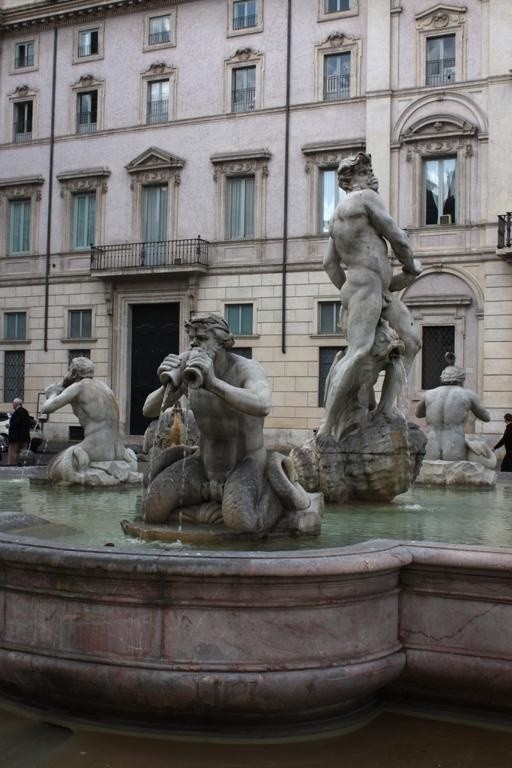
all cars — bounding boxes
[0,410,45,452]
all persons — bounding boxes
[414,364,491,461]
[315,151,424,435]
[141,311,285,533]
[40,356,137,468]
[6,397,31,465]
[491,412,512,472]
